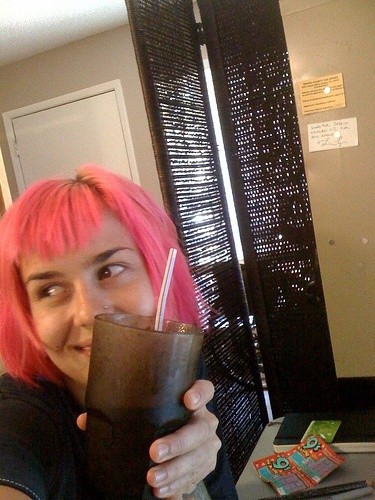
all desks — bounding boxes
[235,415,375,500]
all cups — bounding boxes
[80,313,205,500]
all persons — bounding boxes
[0,165,240,500]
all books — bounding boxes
[272,409,375,453]
